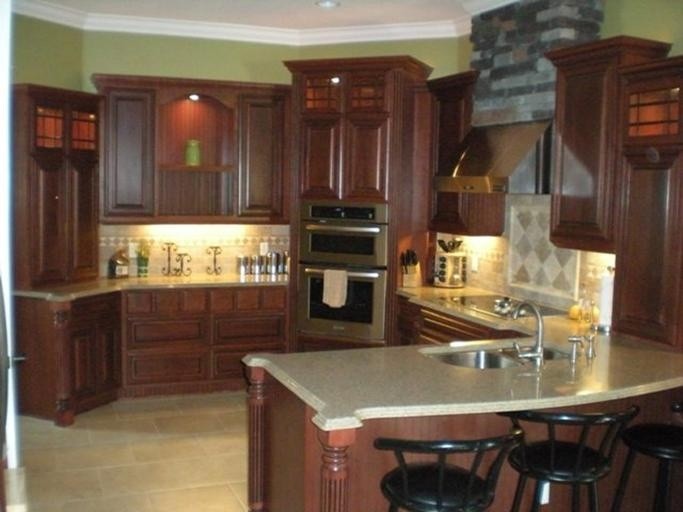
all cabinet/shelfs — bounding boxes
[236,80,286,225]
[207,288,292,397]
[607,54,683,352]
[426,71,504,238]
[123,290,205,401]
[281,53,436,204]
[155,76,236,225]
[90,72,153,225]
[14,291,123,430]
[412,307,519,347]
[549,36,675,256]
[13,82,102,292]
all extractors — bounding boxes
[430,116,556,196]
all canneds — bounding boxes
[236,251,289,274]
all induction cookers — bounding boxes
[438,293,570,321]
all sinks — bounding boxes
[501,345,579,368]
[430,348,524,370]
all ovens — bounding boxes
[294,199,389,339]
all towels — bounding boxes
[321,267,350,311]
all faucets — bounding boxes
[513,299,545,368]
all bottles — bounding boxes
[136,239,149,278]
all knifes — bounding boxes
[399,248,419,266]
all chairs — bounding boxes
[373,422,523,510]
[498,405,640,511]
[613,400,682,511]
